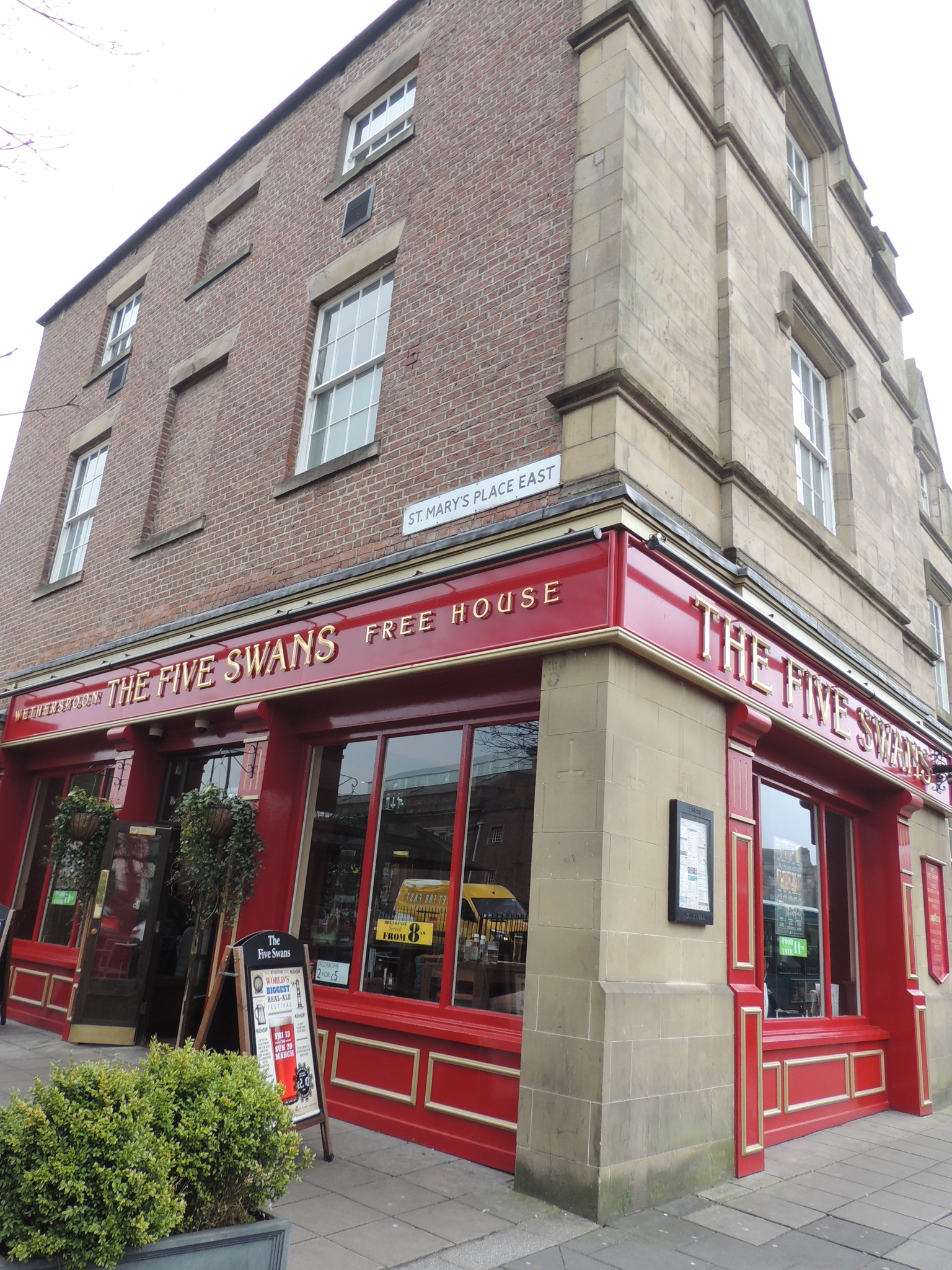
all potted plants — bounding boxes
[166,754,270,933]
[38,766,120,934]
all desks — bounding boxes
[420,954,526,1014]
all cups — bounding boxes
[487,941,500,963]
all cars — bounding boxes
[329,907,395,943]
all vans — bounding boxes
[390,879,527,956]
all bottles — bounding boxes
[465,933,486,964]
